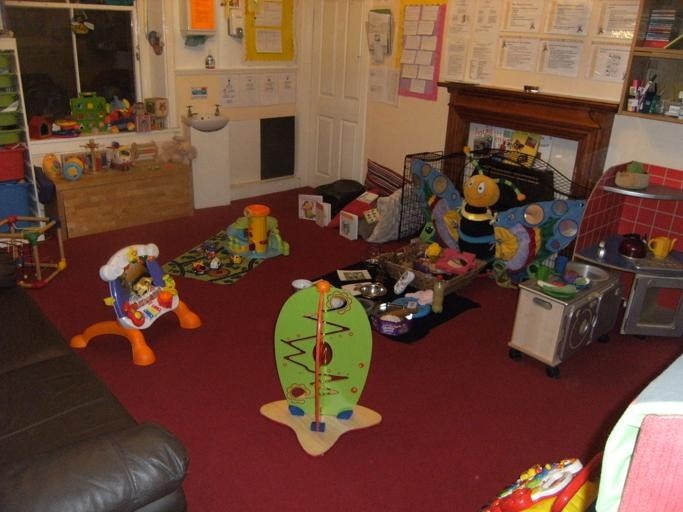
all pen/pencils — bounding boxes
[632,72,657,113]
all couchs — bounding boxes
[0,249,190,511]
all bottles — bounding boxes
[431,275,445,314]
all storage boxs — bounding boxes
[0,52,33,234]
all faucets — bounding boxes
[214,103,220,116]
[186,105,198,117]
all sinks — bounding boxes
[181,112,229,132]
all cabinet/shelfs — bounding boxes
[0,36,45,248]
[615,1,683,124]
[571,160,683,341]
[41,157,193,242]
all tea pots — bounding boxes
[617,233,648,258]
[648,236,678,261]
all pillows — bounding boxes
[327,158,420,244]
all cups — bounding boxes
[536,266,550,281]
[554,255,569,273]
